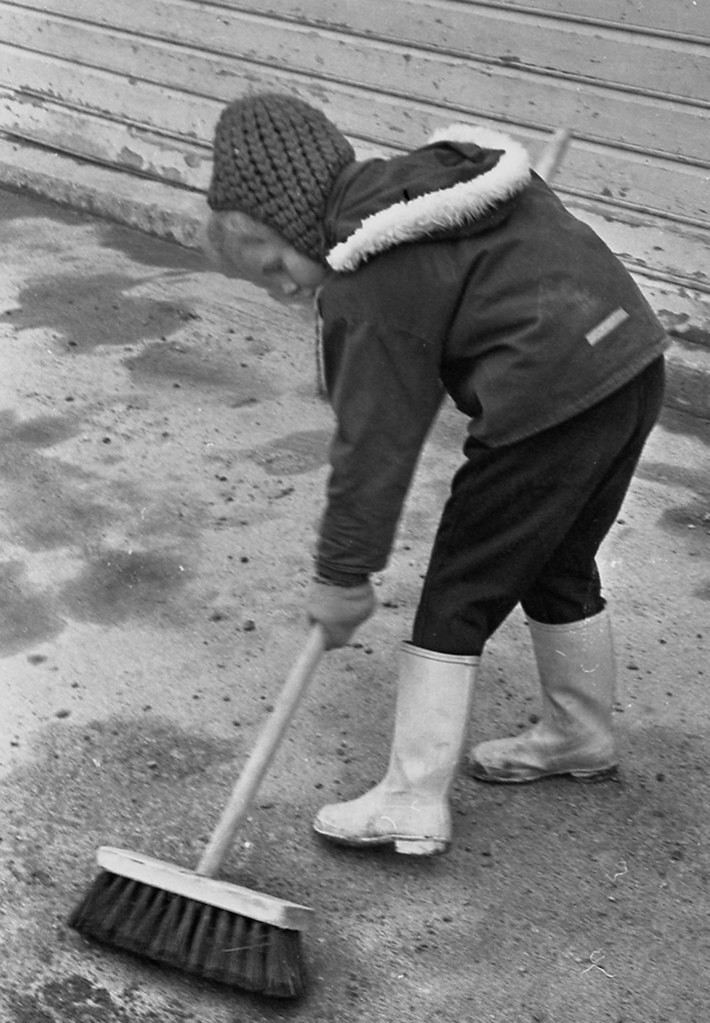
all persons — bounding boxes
[208,92,671,858]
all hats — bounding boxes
[206,93,356,267]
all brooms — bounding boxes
[57,125,581,1000]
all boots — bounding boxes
[462,612,620,781]
[311,643,482,854]
